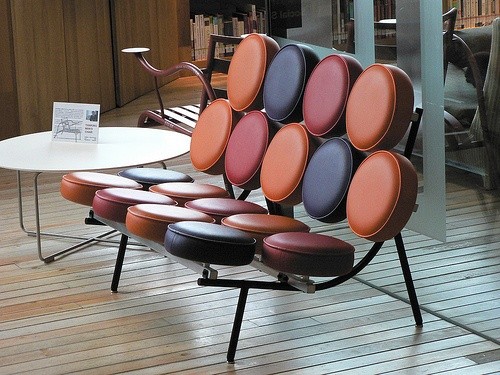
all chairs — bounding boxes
[120,33,244,138]
[344,7,500,188]
[442,7,500,189]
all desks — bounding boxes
[0,126,193,263]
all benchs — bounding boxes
[59,34,423,365]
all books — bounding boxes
[331,0,396,45]
[442,0,500,29]
[190,4,267,61]
